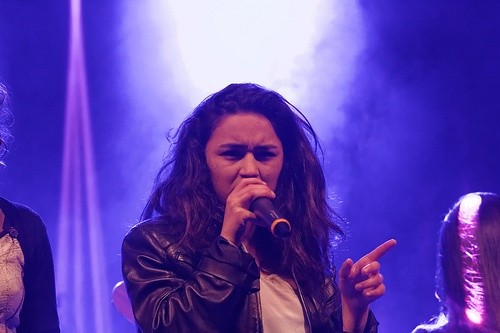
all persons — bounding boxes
[409,189,500,333]
[0,82,65,333]
[121,82,399,333]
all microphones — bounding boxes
[249,197,292,239]
